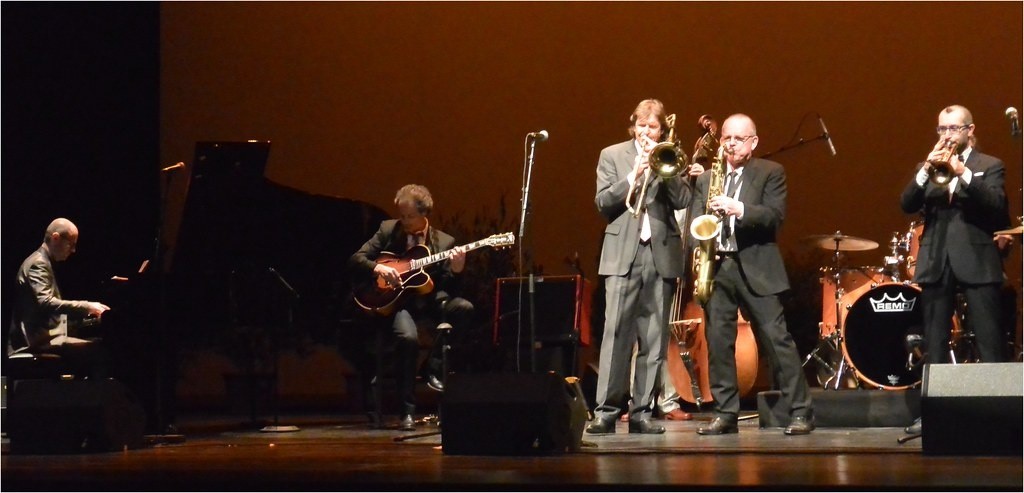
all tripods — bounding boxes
[802,239,862,389]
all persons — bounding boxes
[586,99,692,433]
[900,106,1009,433]
[8,217,111,368]
[620,163,704,422]
[690,113,816,435]
[350,183,473,431]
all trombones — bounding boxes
[625,114,690,218]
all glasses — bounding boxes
[720,136,753,146]
[936,124,969,135]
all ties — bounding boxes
[413,234,422,246]
[721,172,737,247]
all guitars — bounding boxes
[350,231,516,312]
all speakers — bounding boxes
[440,371,589,456]
[7,377,146,455]
[493,276,595,347]
[920,361,1023,457]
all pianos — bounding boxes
[67,141,406,427]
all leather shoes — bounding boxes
[586,416,616,433]
[628,418,666,434]
[415,366,445,392]
[399,414,416,431]
[621,413,629,421]
[697,416,738,435]
[665,408,692,420]
[905,420,921,434]
[785,417,815,435]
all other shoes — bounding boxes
[700,397,718,412]
[678,397,699,413]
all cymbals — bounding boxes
[799,234,879,251]
[993,226,1024,234]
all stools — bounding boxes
[340,314,397,429]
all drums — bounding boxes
[820,264,895,346]
[906,220,925,278]
[840,281,959,392]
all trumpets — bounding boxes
[928,138,960,185]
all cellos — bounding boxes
[668,113,758,408]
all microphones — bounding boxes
[1006,107,1019,135]
[160,162,186,175]
[817,114,837,155]
[528,130,549,140]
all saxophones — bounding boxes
[690,142,735,302]
